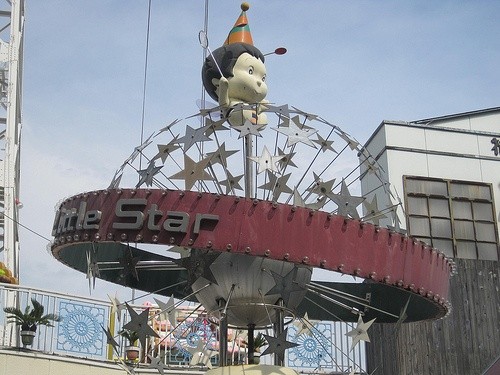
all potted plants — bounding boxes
[117,330,140,360]
[241,331,268,365]
[2,298,64,346]
